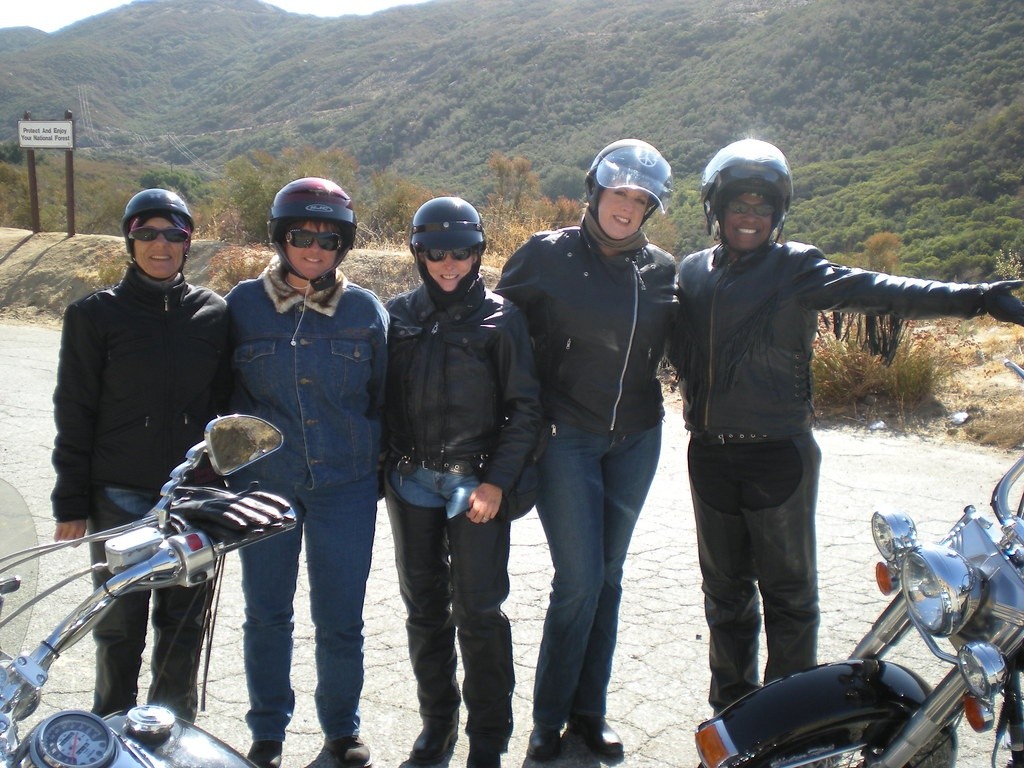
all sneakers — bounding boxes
[324,736,371,768]
[247,740,283,768]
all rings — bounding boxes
[483,516,489,522]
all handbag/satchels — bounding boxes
[497,456,540,520]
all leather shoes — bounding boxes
[467,751,500,768]
[410,710,459,764]
[527,724,561,760]
[568,714,622,755]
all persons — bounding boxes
[490,138,680,761]
[222,177,391,768]
[49,188,234,727]
[665,136,1023,725]
[381,194,546,768]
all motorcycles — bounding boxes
[695,452,1024,768]
[0,411,295,768]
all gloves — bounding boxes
[977,280,1024,327]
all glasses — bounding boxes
[285,229,343,251]
[426,249,470,260]
[128,227,189,242]
[726,200,775,216]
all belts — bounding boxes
[718,432,770,441]
[412,455,489,474]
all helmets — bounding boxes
[120,189,194,253]
[701,139,793,225]
[585,138,672,213]
[411,197,483,250]
[270,177,357,250]
